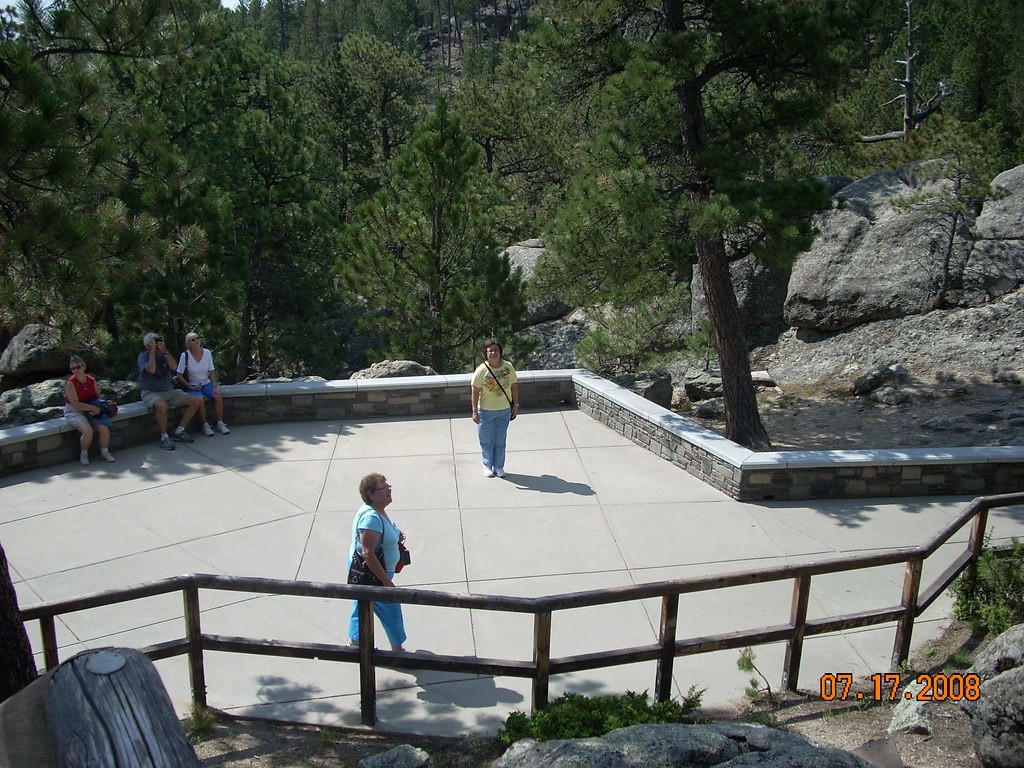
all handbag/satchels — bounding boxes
[510,402,516,421]
[347,509,385,587]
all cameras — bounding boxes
[155,336,164,343]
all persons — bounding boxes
[176,332,230,436]
[471,339,518,477]
[345,473,407,650]
[138,333,200,451]
[63,355,115,465]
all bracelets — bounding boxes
[515,403,519,406]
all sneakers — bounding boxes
[483,464,494,477]
[159,437,176,451]
[203,425,214,437]
[172,431,194,443]
[80,453,89,466]
[217,424,230,434]
[495,466,506,478]
[101,452,116,463]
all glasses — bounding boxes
[190,337,200,342]
[70,364,84,371]
[486,350,500,354]
